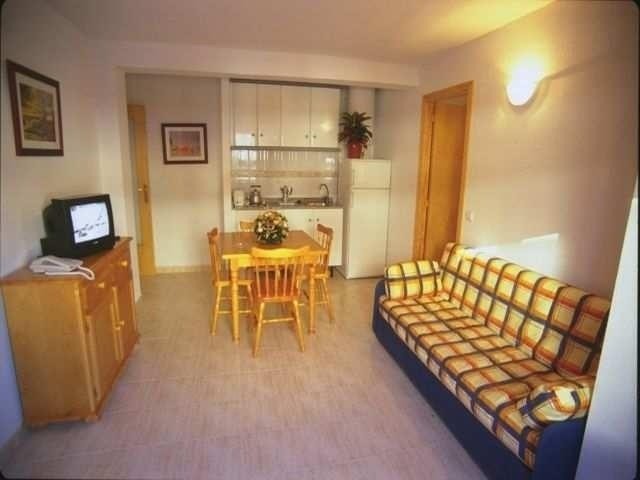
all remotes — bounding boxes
[114,236,120,241]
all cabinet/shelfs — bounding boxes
[232,210,287,232]
[287,209,344,277]
[1,237,140,431]
[230,83,279,148]
[278,85,342,151]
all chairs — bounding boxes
[206,227,253,335]
[290,224,335,332]
[240,222,286,278]
[248,245,310,357]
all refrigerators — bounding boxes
[335,158,393,280]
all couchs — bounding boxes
[373,243,611,480]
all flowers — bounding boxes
[255,211,289,242]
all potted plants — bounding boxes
[336,111,372,158]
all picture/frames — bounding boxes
[5,59,64,156]
[162,122,208,164]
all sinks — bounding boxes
[303,197,328,207]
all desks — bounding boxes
[219,231,328,343]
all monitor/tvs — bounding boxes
[43,194,115,258]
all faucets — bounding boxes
[319,183,330,205]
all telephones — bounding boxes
[29,255,84,274]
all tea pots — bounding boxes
[247,185,262,204]
